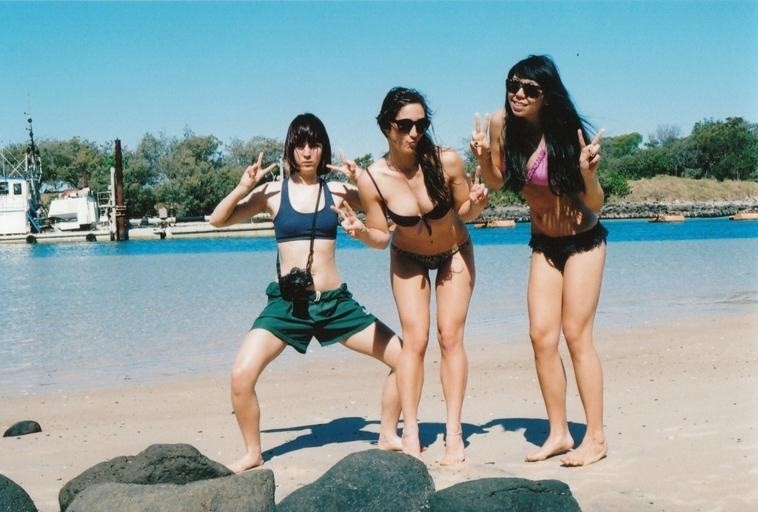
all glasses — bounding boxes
[388,118,431,134]
[506,79,544,98]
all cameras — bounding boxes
[279,267,316,302]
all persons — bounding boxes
[209,109,406,475]
[466,50,610,468]
[328,85,489,466]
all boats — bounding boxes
[474,219,516,229]
[0,112,276,244]
[649,210,685,223]
[730,205,758,220]
